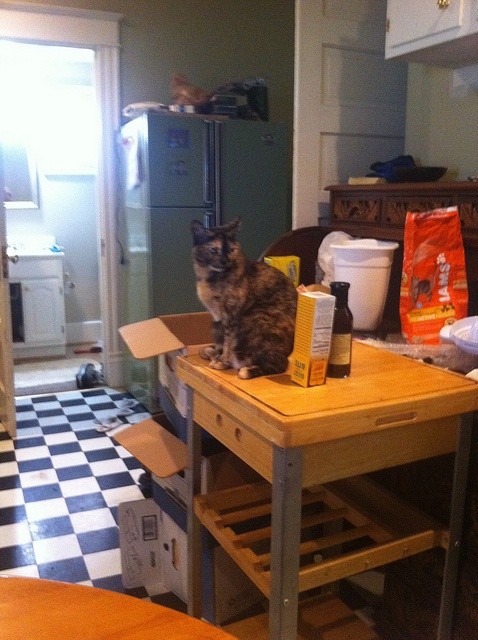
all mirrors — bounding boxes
[2,141,40,209]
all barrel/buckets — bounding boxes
[328,238,399,331]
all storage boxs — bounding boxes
[115,498,263,625]
[113,416,266,535]
[117,311,215,443]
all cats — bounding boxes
[189,218,298,380]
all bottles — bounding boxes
[324,282,352,379]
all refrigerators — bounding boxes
[118,114,289,414]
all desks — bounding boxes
[0,573,238,640]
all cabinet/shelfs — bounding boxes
[9,253,66,347]
[176,338,477,640]
[381,0,478,60]
